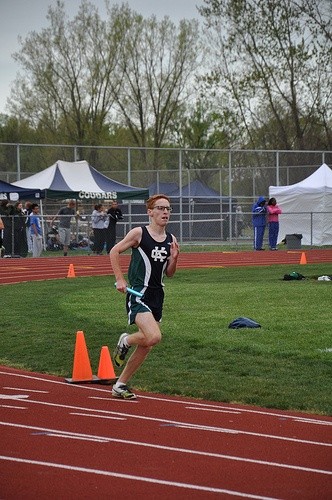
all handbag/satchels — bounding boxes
[228,318,261,329]
[284,271,302,280]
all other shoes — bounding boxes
[256,248,265,250]
[271,248,278,250]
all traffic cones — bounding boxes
[64,330,100,384]
[95,345,119,383]
[67,263,77,278]
[300,252,308,265]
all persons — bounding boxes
[109,194,181,400]
[106,199,123,254]
[91,204,109,255]
[51,200,79,256]
[252,196,267,251]
[0,200,44,257]
[266,197,283,250]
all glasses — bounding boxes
[152,205,173,212]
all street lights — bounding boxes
[228,144,242,242]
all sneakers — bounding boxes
[112,332,130,368]
[111,384,136,399]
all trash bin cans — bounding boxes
[286,234,302,249]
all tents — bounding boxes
[10,160,150,254]
[269,162,332,246]
[116,180,236,240]
[0,179,47,257]
